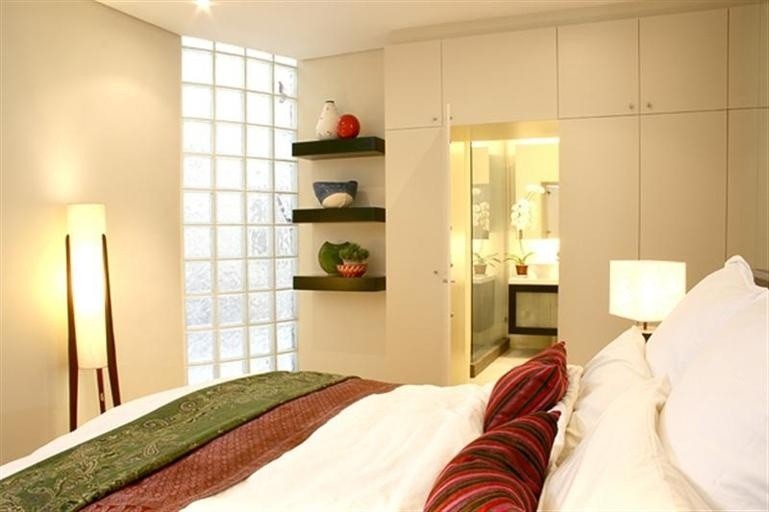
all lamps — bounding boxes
[606,253,690,333]
[506,181,552,250]
[62,200,125,432]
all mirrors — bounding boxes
[515,177,561,267]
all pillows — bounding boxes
[658,291,768,510]
[547,323,675,464]
[421,405,567,511]
[478,337,573,437]
[536,368,678,509]
[645,254,767,387]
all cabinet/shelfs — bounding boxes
[729,3,769,109]
[560,115,638,372]
[559,6,729,120]
[382,37,444,131]
[638,112,728,296]
[473,279,495,333]
[445,24,559,125]
[292,135,384,293]
[505,279,558,336]
[728,109,769,282]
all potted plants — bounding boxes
[506,248,536,276]
[473,250,503,277]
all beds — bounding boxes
[0,268,769,510]
